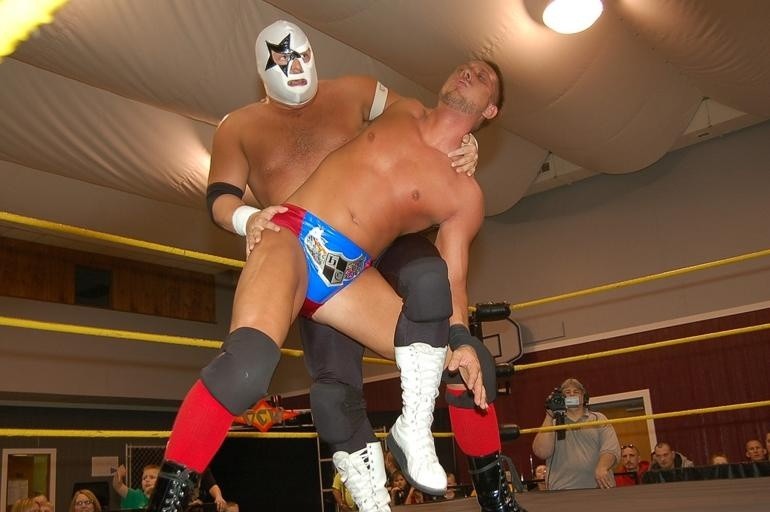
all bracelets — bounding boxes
[232,204,262,237]
[447,322,473,353]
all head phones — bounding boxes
[584,391,589,403]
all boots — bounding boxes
[465,451,528,512]
[148,459,201,512]
[332,442,392,512]
[387,343,448,496]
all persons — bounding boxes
[205,19,530,511]
[710,452,728,467]
[532,464,548,492]
[471,448,518,497]
[745,438,769,461]
[146,58,505,512]
[647,442,694,472]
[764,431,770,460]
[12,460,239,512]
[532,377,621,491]
[331,448,467,511]
[614,444,651,487]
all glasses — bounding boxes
[621,445,633,449]
[75,500,91,505]
